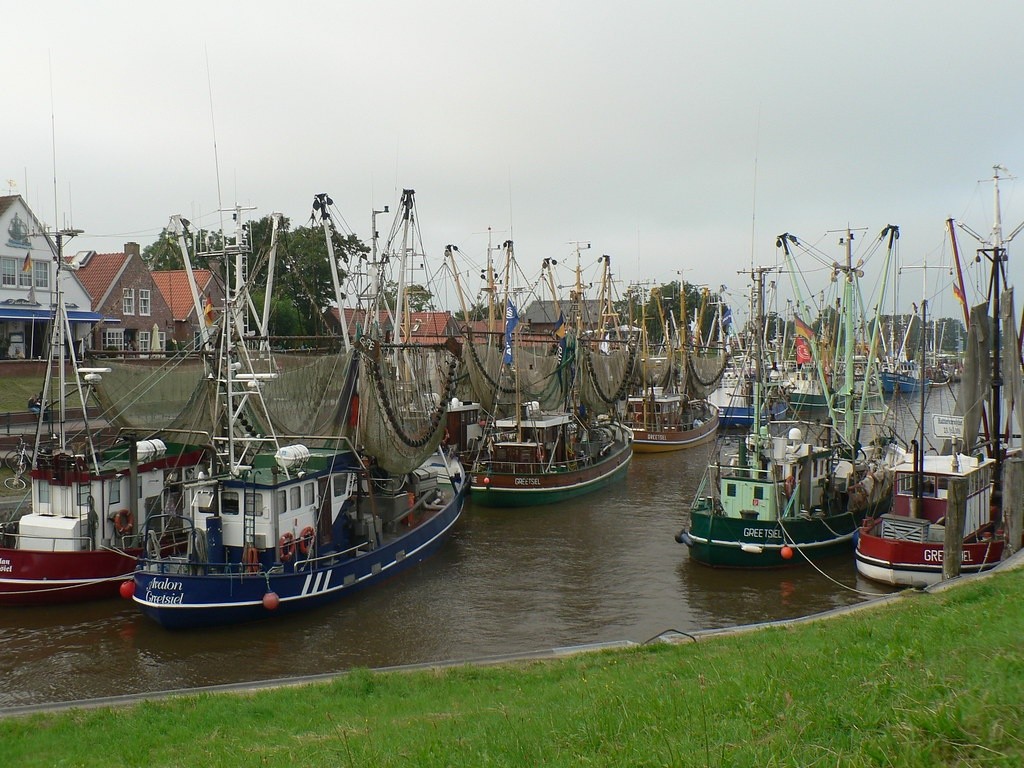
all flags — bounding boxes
[720,308,733,328]
[552,309,567,368]
[203,291,215,329]
[503,299,520,365]
[792,313,815,341]
[952,281,963,305]
[795,335,811,366]
[22,250,34,274]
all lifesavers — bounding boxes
[536,445,545,461]
[299,525,317,554]
[441,427,449,443]
[113,508,135,533]
[279,531,297,561]
[784,475,796,495]
[633,413,640,423]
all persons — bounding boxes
[27,392,40,421]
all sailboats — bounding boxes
[0,164,1024,632]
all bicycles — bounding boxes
[4,433,59,491]
